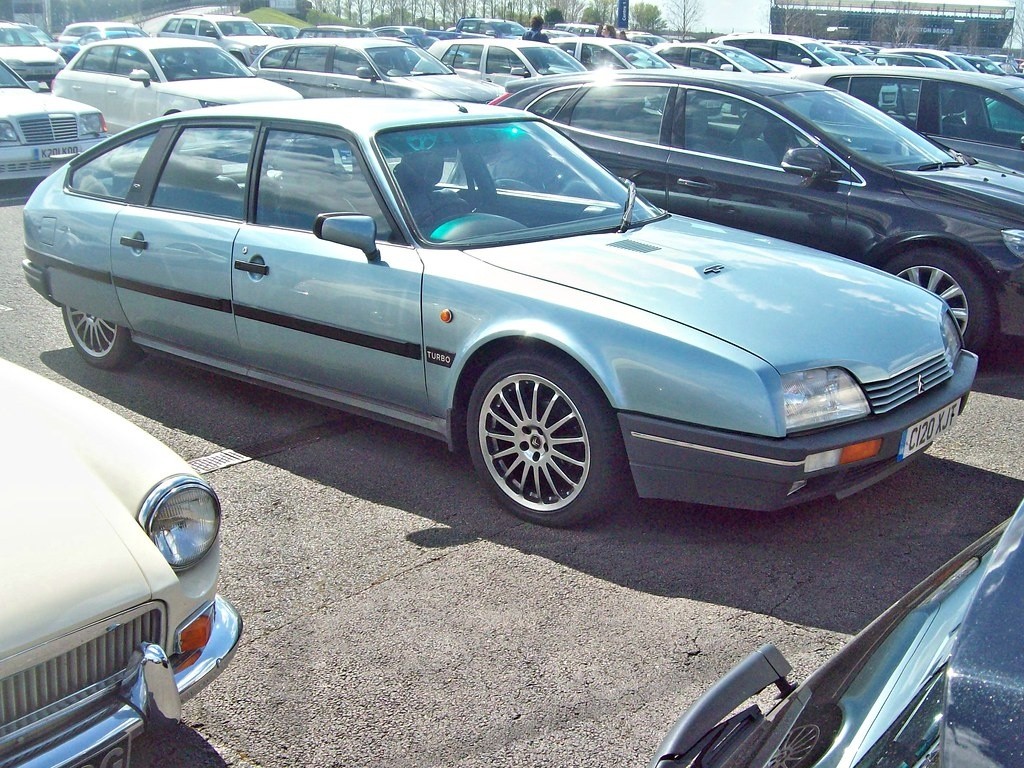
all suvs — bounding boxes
[155,13,287,73]
[0,19,67,91]
[3,21,81,63]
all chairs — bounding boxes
[84,51,198,79]
[174,22,234,39]
[448,52,558,75]
[572,47,638,71]
[569,95,779,167]
[284,51,397,79]
[670,49,747,72]
[861,90,969,139]
[124,143,471,245]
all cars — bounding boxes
[0,357,246,763]
[258,17,1024,99]
[650,481,1024,768]
[56,21,152,46]
[250,36,507,111]
[450,69,1024,369]
[22,98,983,529]
[766,65,1024,173]
[0,57,110,182]
[49,37,304,152]
[58,31,172,65]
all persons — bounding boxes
[522,15,550,71]
[595,25,629,40]
